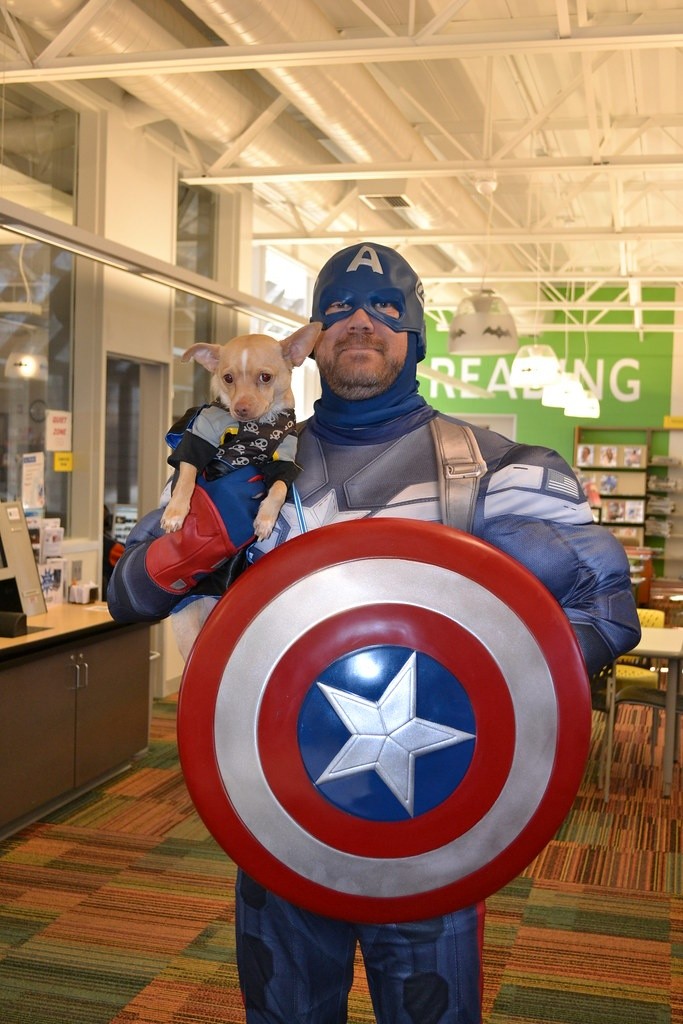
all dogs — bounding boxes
[159,322,323,543]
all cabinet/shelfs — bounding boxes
[0,625,152,844]
[573,425,683,593]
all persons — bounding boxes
[101,241,643,1024]
[100,504,126,579]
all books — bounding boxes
[646,453,677,537]
[24,517,65,605]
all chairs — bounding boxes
[599,686,682,789]
[592,608,665,769]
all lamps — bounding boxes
[509,253,600,419]
[448,175,519,357]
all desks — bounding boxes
[597,627,682,803]
